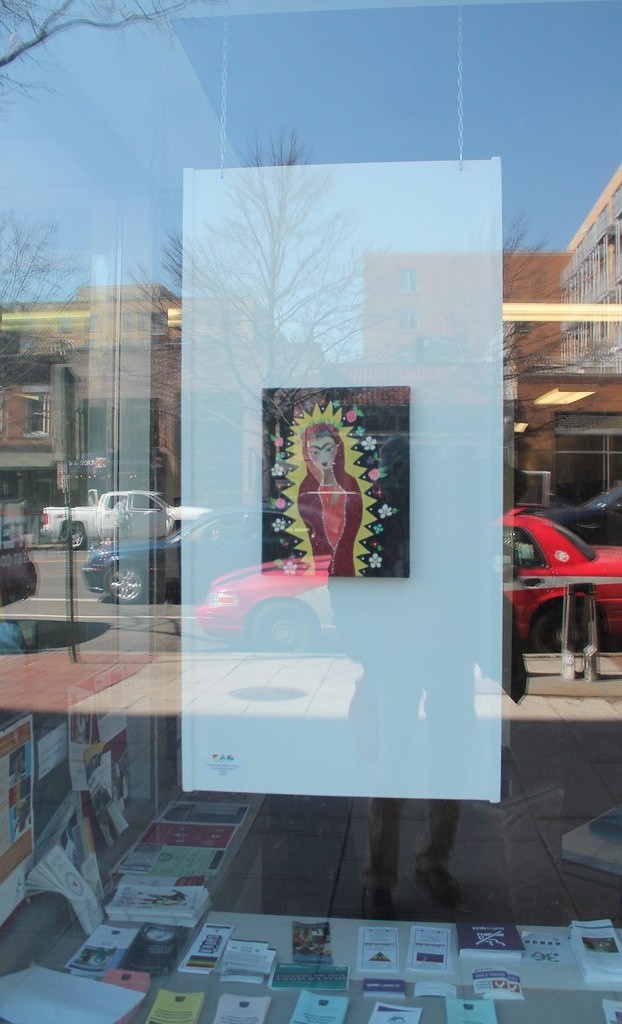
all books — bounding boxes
[63,883,213,976]
[566,919,622,983]
[219,937,277,984]
[456,916,526,960]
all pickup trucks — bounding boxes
[37,484,213,551]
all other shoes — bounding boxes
[415,861,465,910]
[361,879,397,920]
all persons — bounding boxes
[112,497,131,540]
[328,432,530,921]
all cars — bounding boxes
[80,507,254,604]
[532,478,621,544]
[192,504,621,654]
